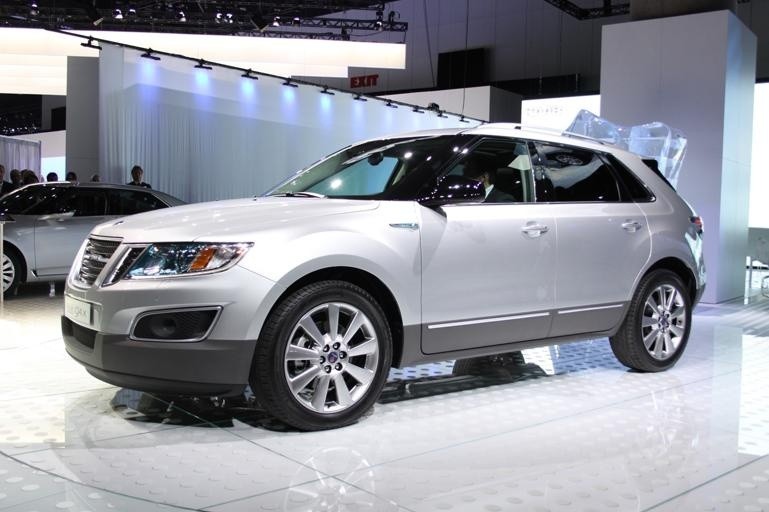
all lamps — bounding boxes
[47,30,488,123]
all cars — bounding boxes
[0,180,189,295]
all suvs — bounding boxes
[60,123,707,431]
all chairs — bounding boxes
[495,166,522,200]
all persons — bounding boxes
[91,173,101,181]
[0,162,39,199]
[127,164,152,189]
[65,171,77,180]
[46,172,58,181]
